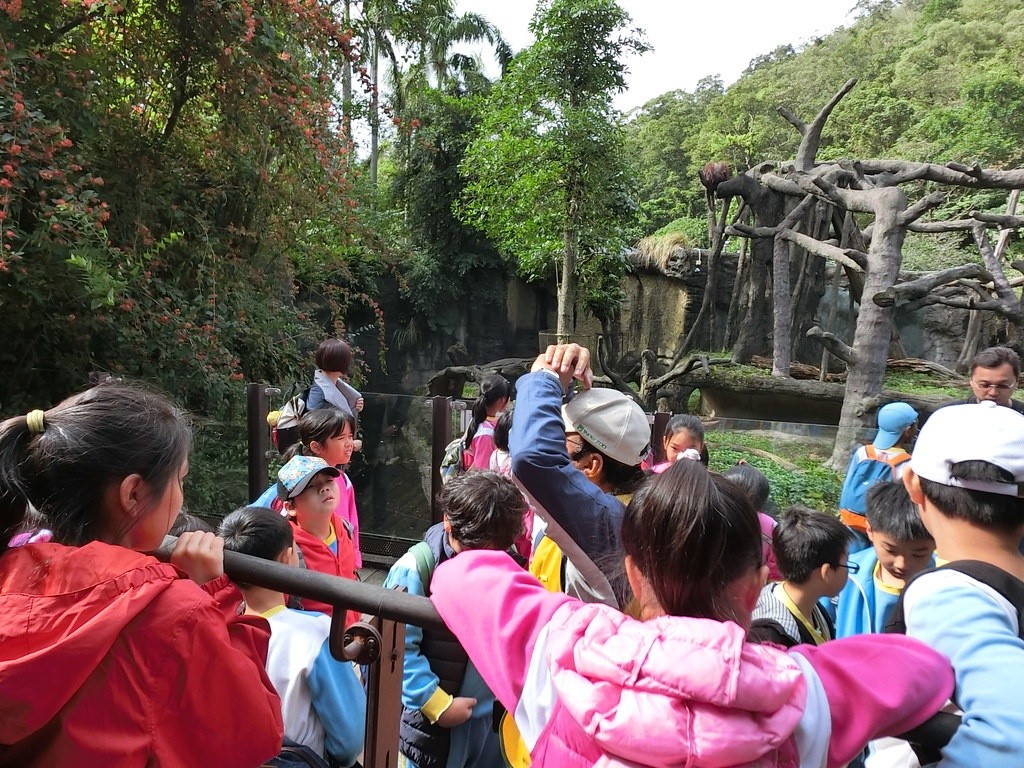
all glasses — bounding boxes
[816,560,860,575]
[970,379,1016,390]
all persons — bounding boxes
[276,456,361,628]
[0,378,283,767]
[894,345,1023,768]
[272,406,361,570]
[305,338,363,451]
[218,504,365,767]
[382,342,955,768]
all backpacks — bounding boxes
[441,417,495,486]
[839,443,912,532]
[267,383,323,456]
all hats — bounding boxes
[562,387,652,467]
[277,455,340,501]
[873,403,917,450]
[911,400,1024,496]
